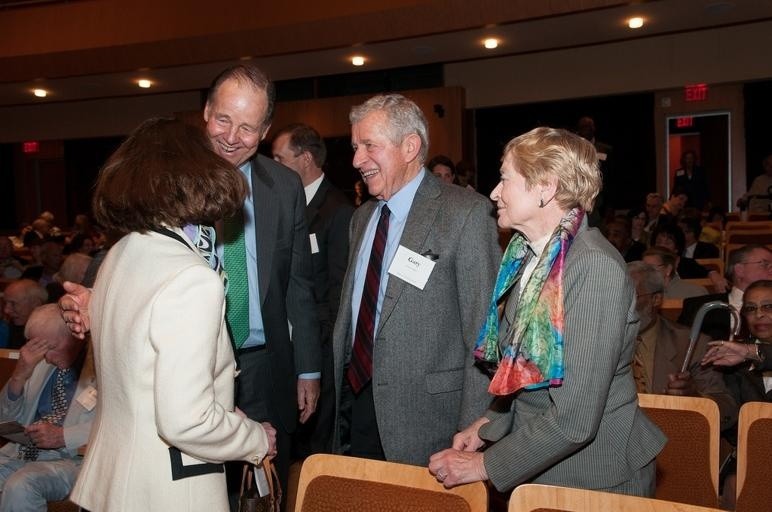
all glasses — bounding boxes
[741,303,772,315]
[743,260,772,271]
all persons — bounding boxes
[58,54,321,512]
[69,117,280,512]
[604,152,771,351]
[354,181,377,209]
[624,261,740,472]
[331,92,504,465]
[427,127,669,497]
[429,155,476,192]
[0,302,85,512]
[270,122,358,456]
[2,211,109,351]
[702,282,771,502]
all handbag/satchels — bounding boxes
[239,480,283,511]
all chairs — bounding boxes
[695,258,725,278]
[657,298,684,323]
[735,401,772,512]
[293,454,489,512]
[685,278,716,296]
[507,483,728,512]
[724,212,772,274]
[0,348,21,391]
[637,393,720,509]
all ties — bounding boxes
[18,368,71,461]
[346,205,390,395]
[223,205,249,350]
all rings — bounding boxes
[437,471,448,478]
